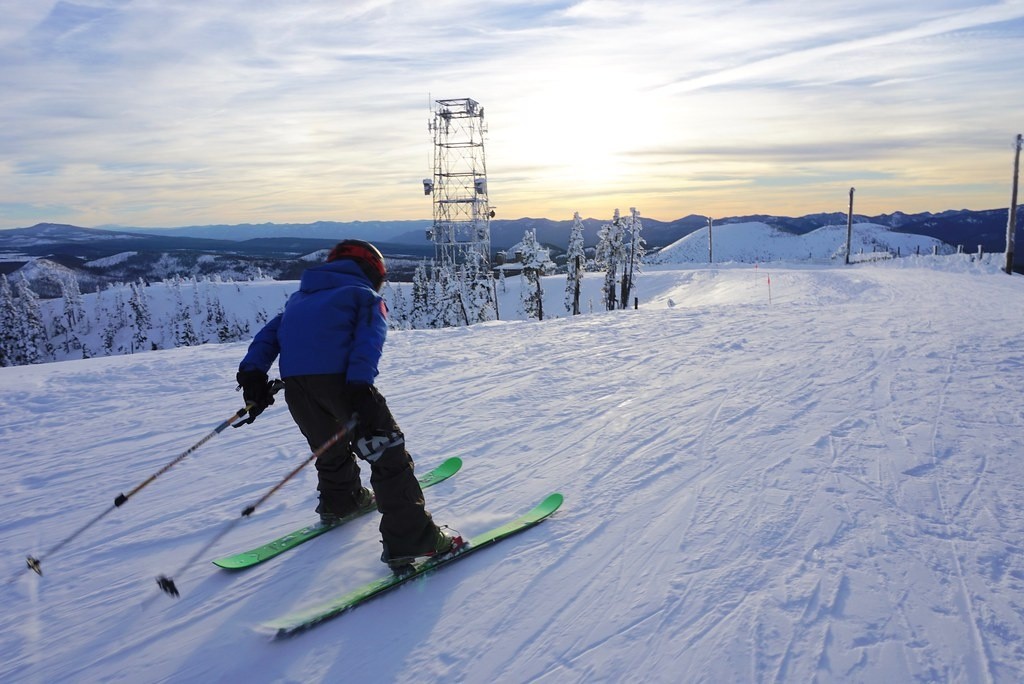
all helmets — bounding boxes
[325,239,386,292]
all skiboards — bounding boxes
[209,455,565,639]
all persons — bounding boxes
[238,239,463,575]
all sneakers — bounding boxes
[315,486,373,525]
[380,526,454,567]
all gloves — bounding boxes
[340,384,382,436]
[232,368,275,428]
